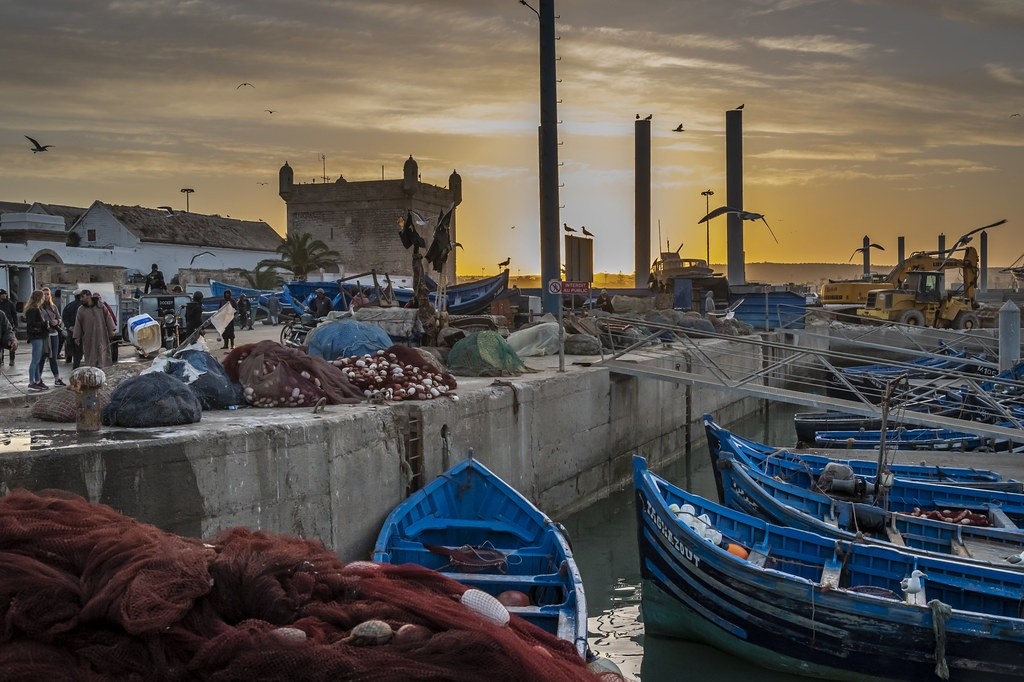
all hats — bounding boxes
[0,288,6,295]
[601,289,607,294]
[315,288,324,294]
[73,289,81,295]
[241,293,246,297]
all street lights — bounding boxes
[181,189,195,213]
[701,190,714,267]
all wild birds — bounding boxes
[697,205,779,243]
[24,134,56,154]
[411,210,432,226]
[581,226,594,237]
[236,82,255,90]
[636,114,640,119]
[645,114,652,121]
[498,257,511,266]
[849,243,885,263]
[256,182,268,186]
[190,251,216,265]
[563,223,578,235]
[736,104,744,109]
[708,297,745,320]
[259,218,264,221]
[672,123,685,132]
[157,206,179,217]
[227,214,230,217]
[264,110,278,114]
[937,218,1008,271]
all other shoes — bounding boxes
[221,346,228,349]
[9,359,16,366]
[55,378,66,386]
[35,379,48,390]
[230,343,234,349]
[58,355,65,359]
[249,327,255,330]
[28,384,42,391]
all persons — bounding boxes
[597,289,613,313]
[23,291,50,390]
[313,288,333,318]
[705,290,715,312]
[144,264,167,294]
[351,289,369,307]
[266,292,281,325]
[0,289,18,366]
[219,289,237,349]
[39,287,66,387]
[184,291,204,344]
[61,289,117,368]
[173,286,183,293]
[238,293,254,330]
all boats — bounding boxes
[629,452,1024,682]
[370,447,589,665]
[701,339,1024,574]
[196,268,519,327]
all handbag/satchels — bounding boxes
[55,325,68,338]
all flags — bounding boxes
[211,303,234,335]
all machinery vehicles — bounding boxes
[819,246,981,330]
